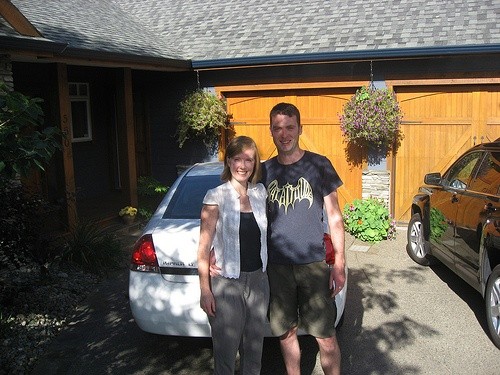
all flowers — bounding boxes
[175,86,229,154]
[337,86,406,150]
[341,194,396,244]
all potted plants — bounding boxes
[118,175,170,224]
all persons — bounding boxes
[259,102,347,375]
[198,136,270,375]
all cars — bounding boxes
[127,161,349,339]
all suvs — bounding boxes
[406,137,500,349]
